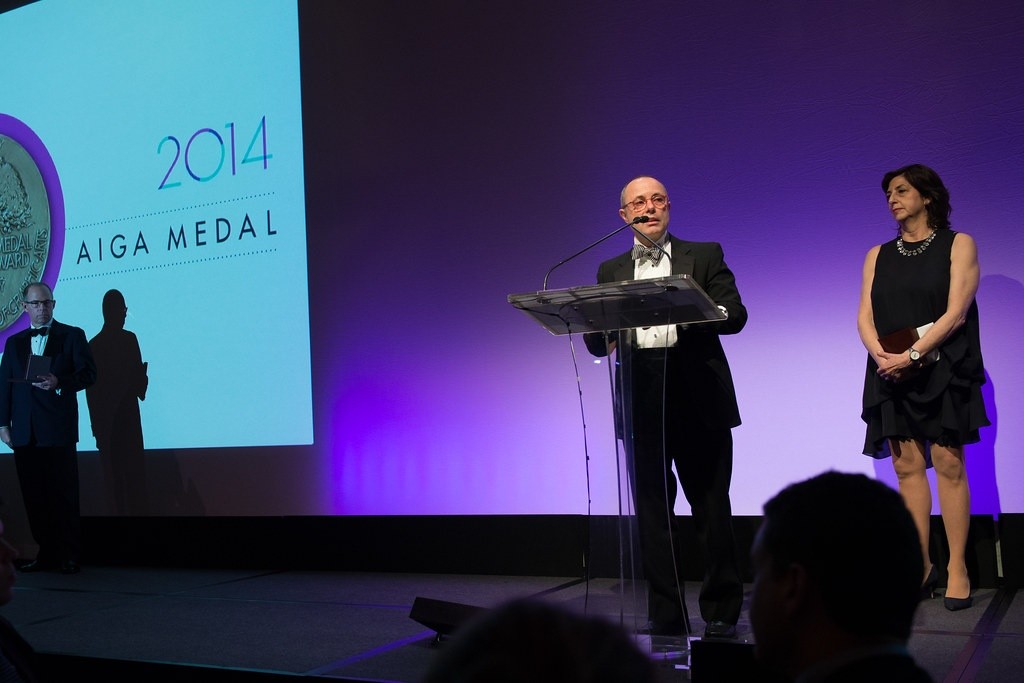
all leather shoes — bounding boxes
[635,612,693,637]
[704,621,739,642]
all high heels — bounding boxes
[944,569,973,611]
[921,563,940,601]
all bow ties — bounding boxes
[631,243,664,264]
[29,326,47,337]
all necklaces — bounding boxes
[897,224,938,255]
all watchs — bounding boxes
[909,347,920,364]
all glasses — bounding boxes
[623,193,669,212]
[24,299,56,309]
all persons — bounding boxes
[858,163,986,611]
[0,281,98,575]
[0,522,37,683]
[432,599,661,683]
[750,470,939,683]
[584,177,747,641]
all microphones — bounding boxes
[544,216,672,291]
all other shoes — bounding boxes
[61,545,84,574]
[22,558,63,572]
